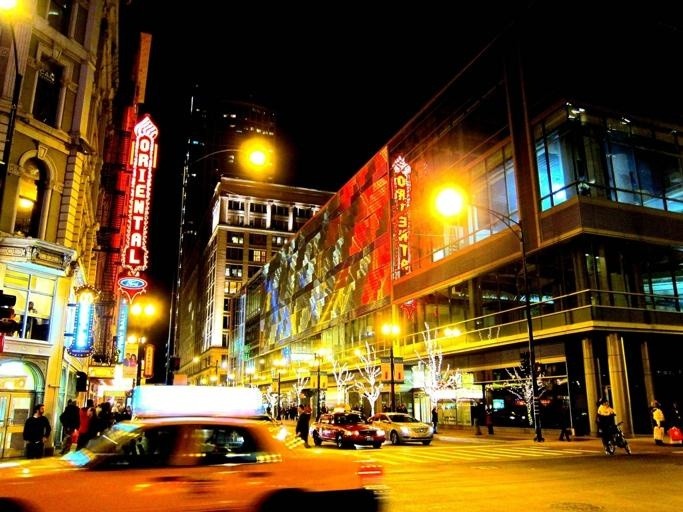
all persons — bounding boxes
[128,354,136,367]
[22,403,52,458]
[265,403,328,421]
[557,404,571,441]
[431,407,439,434]
[122,353,130,367]
[650,400,665,445]
[17,301,36,339]
[378,402,407,416]
[58,398,132,457]
[595,399,622,456]
[294,405,310,447]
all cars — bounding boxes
[470,405,587,430]
[310,403,386,449]
[0,385,390,512]
[365,412,433,446]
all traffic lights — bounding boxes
[517,350,530,377]
[0,289,17,333]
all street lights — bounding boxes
[0,0,24,225]
[164,133,277,385]
[432,185,542,443]
[128,300,157,388]
[378,321,405,411]
[196,356,285,421]
[307,345,332,424]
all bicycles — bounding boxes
[602,420,632,456]
